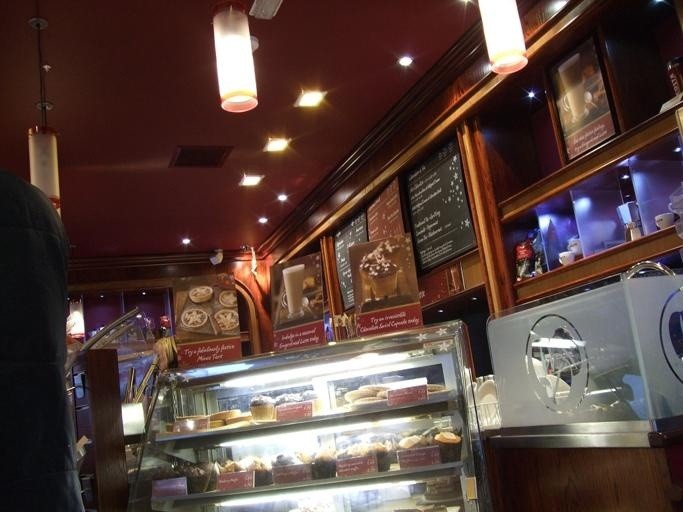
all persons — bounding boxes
[0,167,88,511]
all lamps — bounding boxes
[122,403,147,436]
[212,0,258,113]
[28,16,61,210]
[478,0,528,75]
[70,297,84,337]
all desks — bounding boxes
[485,446,683,512]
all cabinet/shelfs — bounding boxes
[66,349,129,512]
[150,390,464,503]
[497,102,683,304]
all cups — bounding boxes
[281,264,307,320]
[359,266,401,302]
[653,212,676,229]
[557,237,582,266]
[557,53,590,124]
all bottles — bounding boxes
[160,316,172,341]
[666,56,683,96]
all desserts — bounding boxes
[151,384,461,494]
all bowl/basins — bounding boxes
[177,439,464,492]
[250,406,275,423]
